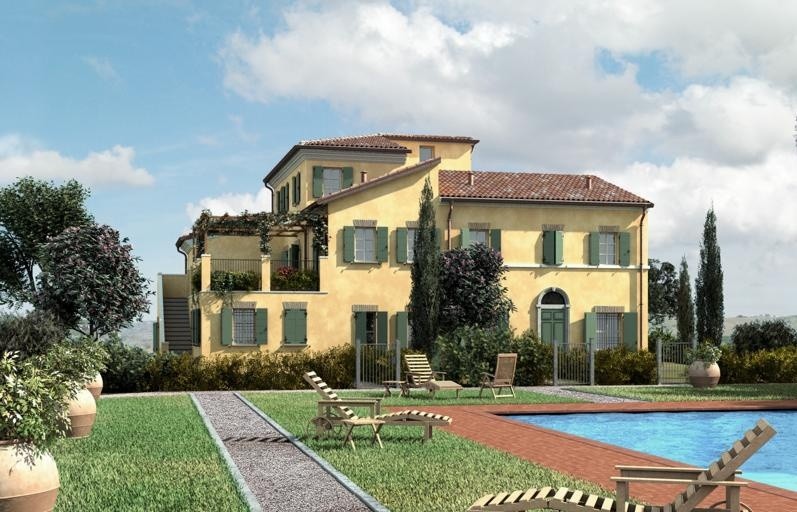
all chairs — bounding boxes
[403,354,464,400]
[466,419,777,512]
[303,371,454,442]
[479,352,518,402]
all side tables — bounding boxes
[336,418,387,453]
[381,380,409,398]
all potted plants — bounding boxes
[682,336,723,389]
[0,336,112,512]
